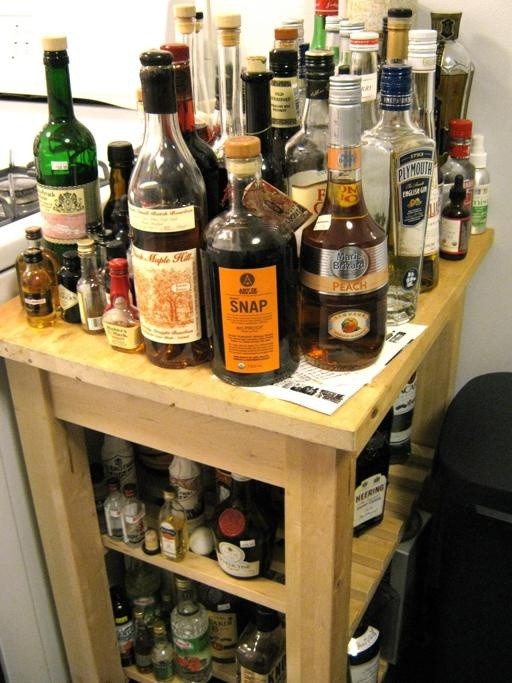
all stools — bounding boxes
[426,369,512,583]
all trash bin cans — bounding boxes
[422,372,512,683]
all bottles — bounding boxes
[99,383,414,682]
[12,0,493,390]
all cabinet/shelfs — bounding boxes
[0,226,492,680]
